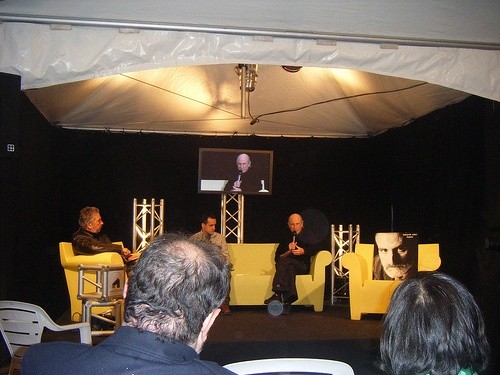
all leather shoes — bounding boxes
[220,304,232,315]
[264,293,282,305]
[281,303,293,314]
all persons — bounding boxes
[264,213,322,313]
[189,214,232,317]
[21,233,238,374]
[372,232,418,281]
[379,270,492,375]
[223,153,263,193]
[72,207,140,268]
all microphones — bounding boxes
[292,231,297,242]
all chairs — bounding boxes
[0,299,93,375]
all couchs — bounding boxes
[219,241,333,313]
[340,242,443,322]
[53,239,140,323]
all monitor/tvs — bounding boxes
[197,148,274,196]
